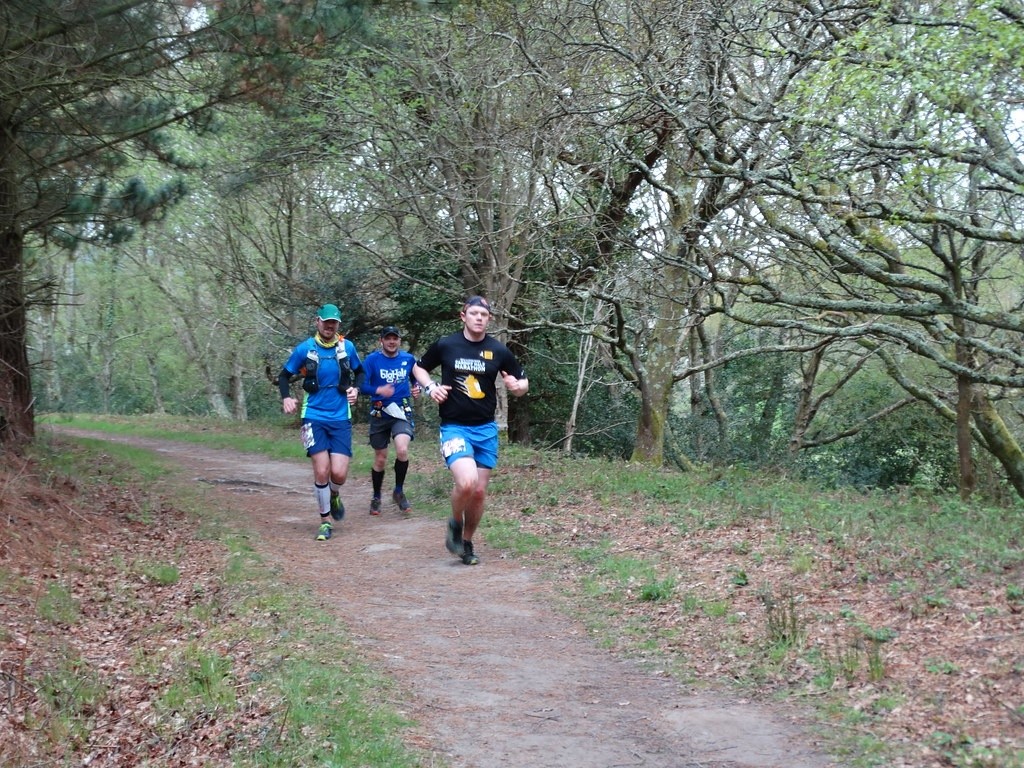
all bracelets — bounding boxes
[418,386,422,393]
[424,381,441,397]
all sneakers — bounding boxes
[393,490,410,512]
[445,521,463,554]
[370,497,382,515]
[317,522,333,540]
[330,494,345,521]
[459,540,480,565]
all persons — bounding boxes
[411,297,529,565]
[361,327,422,517]
[278,304,367,542]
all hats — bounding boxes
[381,326,400,339]
[316,304,342,323]
[463,295,490,316]
